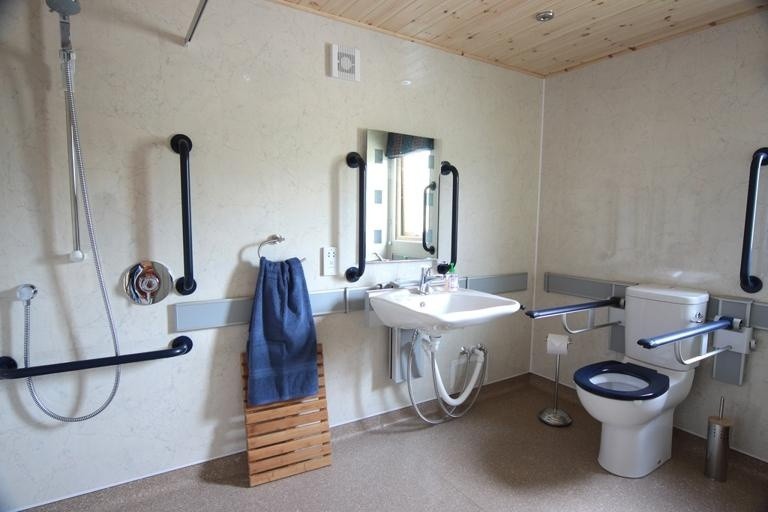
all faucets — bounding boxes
[420,266,445,293]
[373,251,383,261]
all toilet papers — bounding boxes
[547,333,569,355]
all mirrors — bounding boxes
[364,128,443,263]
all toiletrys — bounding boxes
[445,263,459,291]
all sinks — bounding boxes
[371,287,520,328]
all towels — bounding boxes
[246,254,319,407]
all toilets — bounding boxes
[573,283,710,477]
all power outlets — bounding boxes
[321,247,344,277]
